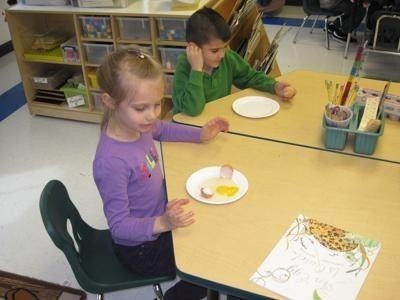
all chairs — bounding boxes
[39,179,177,300]
[292,1,400,83]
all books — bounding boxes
[31,89,66,106]
[228,1,292,75]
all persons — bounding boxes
[302,0,368,45]
[171,6,297,118]
[93,46,230,300]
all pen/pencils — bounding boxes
[376,80,392,119]
[323,80,360,106]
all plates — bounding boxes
[186,166,248,205]
[232,96,279,119]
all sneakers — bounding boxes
[323,23,337,34]
[332,31,356,43]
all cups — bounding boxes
[325,106,353,126]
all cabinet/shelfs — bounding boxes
[5,1,237,127]
